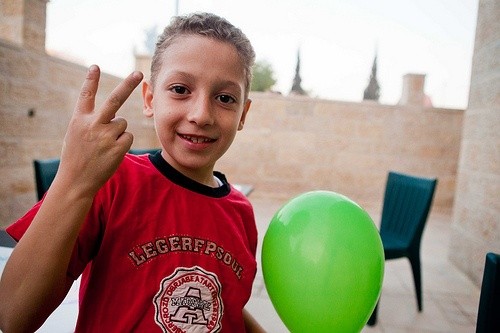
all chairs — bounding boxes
[367,171,437,326]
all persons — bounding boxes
[0,10,268,333]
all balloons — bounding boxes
[261,191,385,333]
